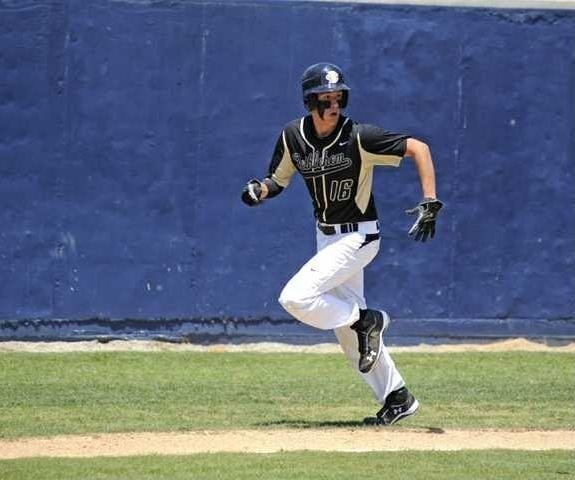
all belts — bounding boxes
[317,221,381,235]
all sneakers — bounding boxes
[363,386,420,426]
[349,306,390,374]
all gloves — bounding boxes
[403,197,443,243]
[242,178,262,206]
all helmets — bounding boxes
[300,62,351,99]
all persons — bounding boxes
[239,59,449,425]
